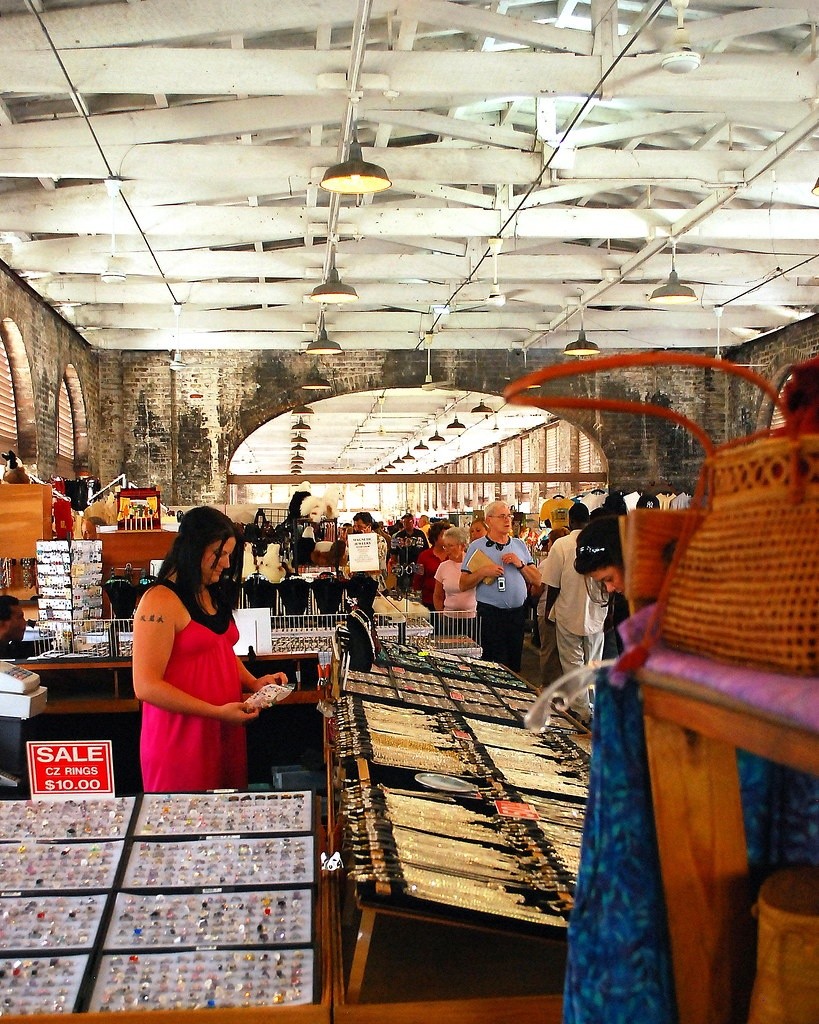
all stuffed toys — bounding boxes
[292,480,346,566]
[242,541,256,578]
[261,543,286,582]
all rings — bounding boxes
[510,558,511,560]
[0,794,304,1015]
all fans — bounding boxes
[602,0,778,88]
[156,303,211,371]
[87,179,136,283]
[422,334,453,391]
[479,238,527,307]
[710,307,767,371]
[376,397,386,436]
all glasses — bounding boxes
[443,544,458,550]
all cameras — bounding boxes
[498,577,505,592]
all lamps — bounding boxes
[289,89,722,475]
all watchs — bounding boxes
[517,560,525,570]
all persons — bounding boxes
[354,512,388,592]
[0,596,27,643]
[471,516,487,541]
[82,516,107,539]
[434,527,477,639]
[390,513,429,589]
[459,501,542,673]
[418,515,430,535]
[579,515,631,658]
[413,521,450,634]
[133,506,288,792]
[374,520,403,540]
[537,492,627,723]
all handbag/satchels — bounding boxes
[503,352,818,685]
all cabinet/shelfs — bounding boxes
[10,653,328,763]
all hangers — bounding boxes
[552,483,673,500]
